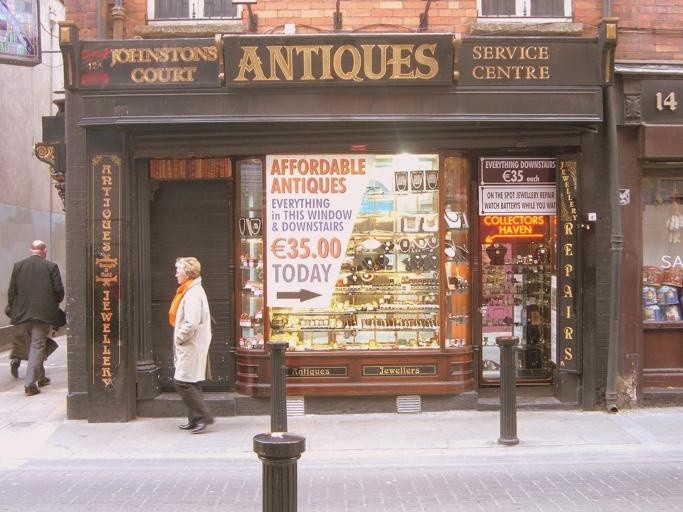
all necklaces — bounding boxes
[239,172,473,352]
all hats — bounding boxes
[174,256,201,278]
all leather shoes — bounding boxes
[24,377,51,396]
[10,358,21,379]
[179,418,214,432]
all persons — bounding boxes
[168,257,215,434]
[5,240,64,396]
